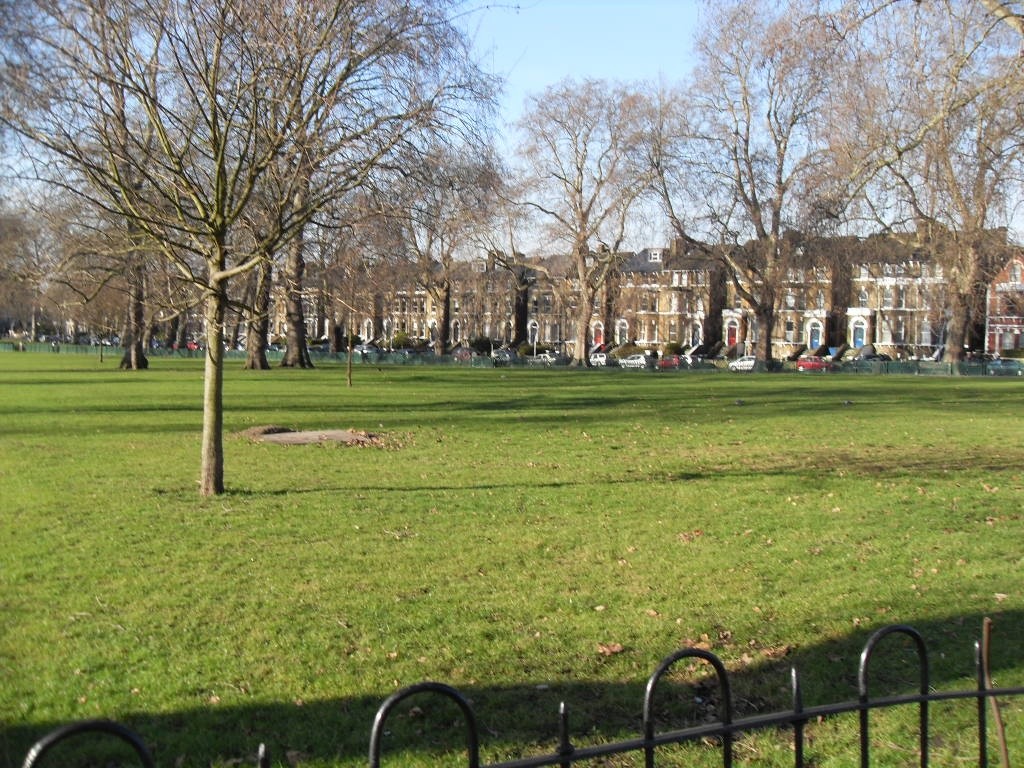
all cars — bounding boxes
[796,355,837,373]
[590,352,613,367]
[728,354,756,373]
[452,346,482,360]
[527,353,565,368]
[492,349,516,368]
[38,333,412,355]
[679,354,704,370]
[617,353,652,370]
[983,358,1024,378]
[654,354,680,370]
